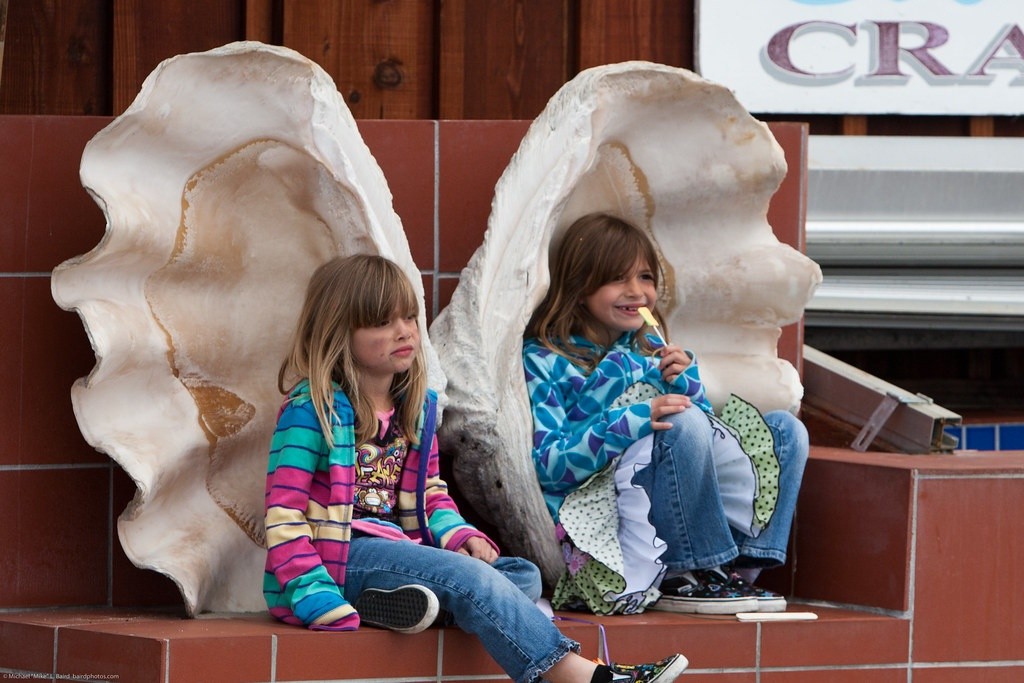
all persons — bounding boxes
[519,211,809,616]
[262,252,689,683]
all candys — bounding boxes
[638,306,659,326]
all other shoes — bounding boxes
[356,584,439,635]
[647,575,761,614]
[591,654,690,683]
[727,570,787,612]
